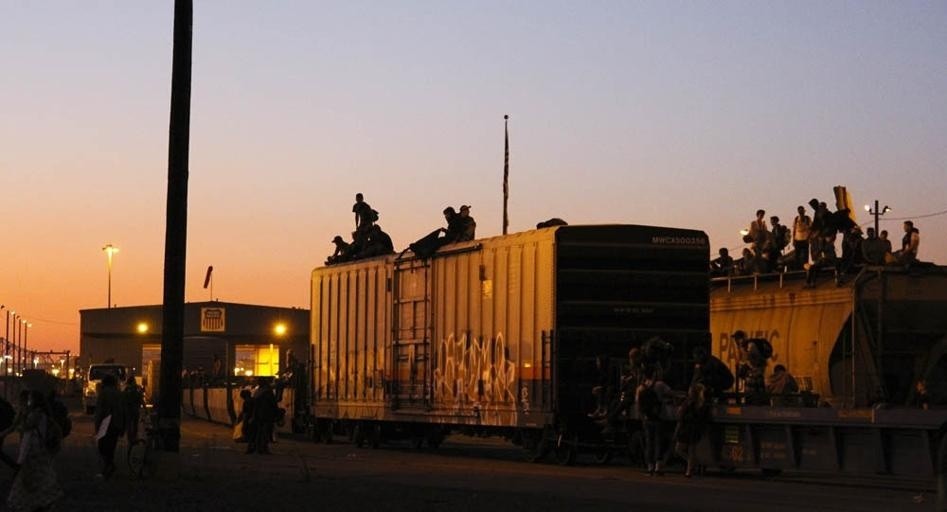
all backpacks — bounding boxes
[370,208,379,222]
[744,338,773,359]
[30,411,61,444]
[638,379,660,417]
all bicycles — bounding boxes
[127,413,163,479]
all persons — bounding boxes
[0,372,152,510]
[206,350,224,387]
[231,378,285,457]
[323,191,478,267]
[582,331,811,479]
[710,198,921,279]
[914,379,932,411]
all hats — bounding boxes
[731,330,745,338]
[459,205,472,215]
[331,236,342,243]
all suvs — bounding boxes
[81,362,137,415]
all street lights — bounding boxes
[740,226,753,240]
[99,243,123,310]
[863,197,894,242]
[0,304,33,378]
